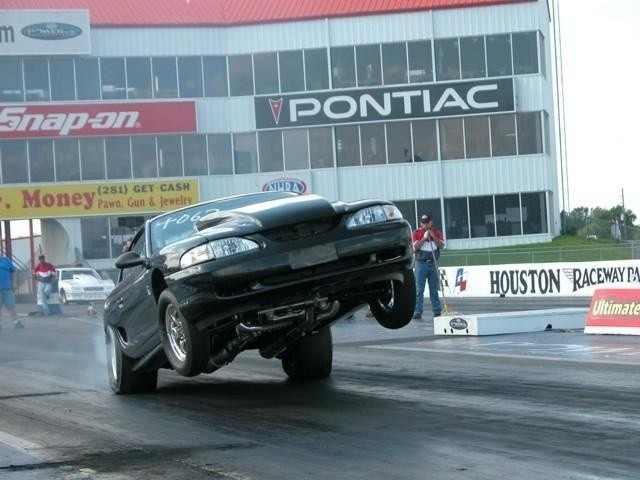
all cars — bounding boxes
[53,265,116,304]
[100,190,417,395]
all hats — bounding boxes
[421,213,433,222]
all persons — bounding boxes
[0,249,25,328]
[33,254,56,316]
[412,214,447,319]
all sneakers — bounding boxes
[412,311,423,320]
